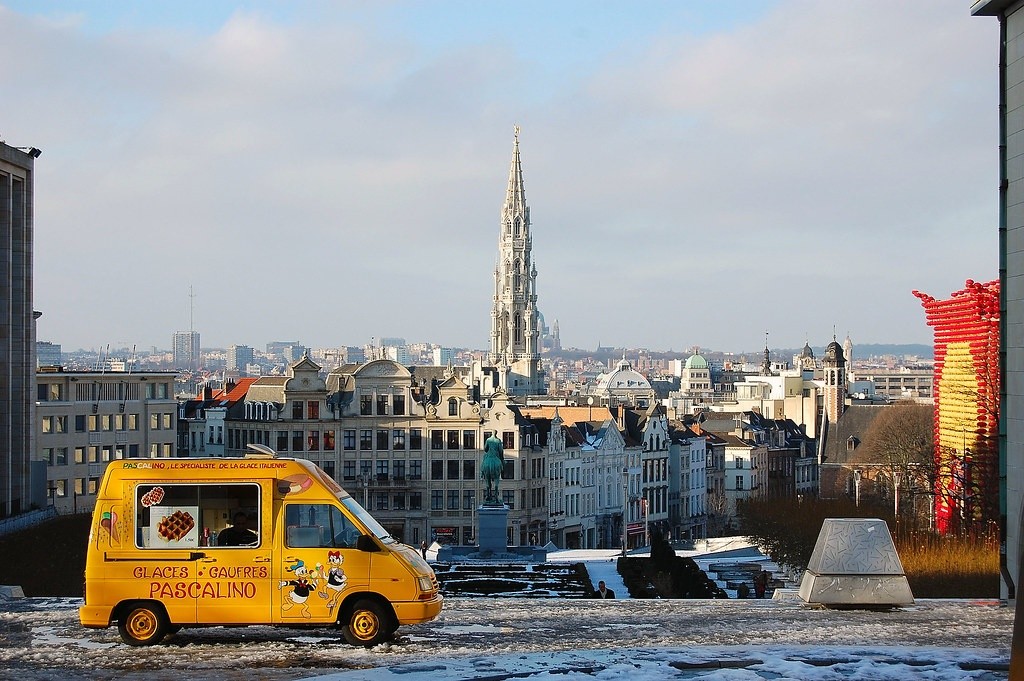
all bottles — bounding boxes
[211,531,217,545]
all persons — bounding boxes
[420,541,428,561]
[218,512,258,546]
[593,581,615,599]
[737,570,769,599]
[480,430,506,479]
[532,534,537,547]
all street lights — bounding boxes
[853,469,863,508]
[929,494,935,530]
[644,499,649,547]
[893,471,902,518]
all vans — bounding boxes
[78,444,445,647]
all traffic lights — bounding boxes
[550,510,564,518]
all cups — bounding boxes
[141,526,150,547]
[204,528,210,537]
